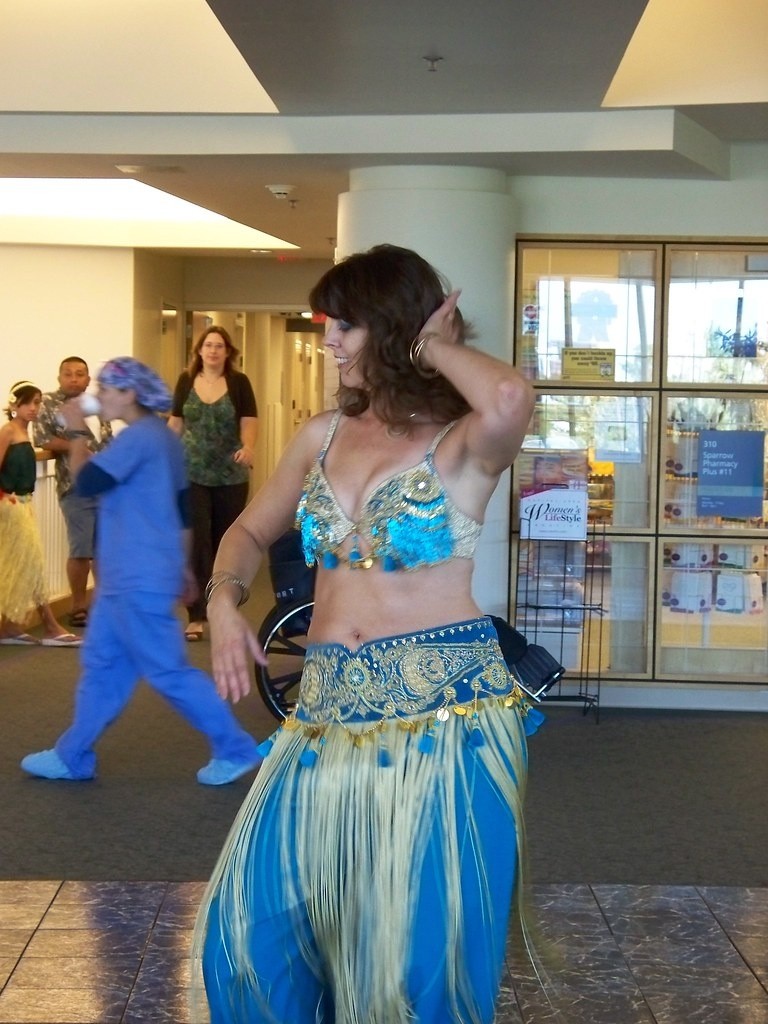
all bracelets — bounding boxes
[205,570,251,607]
[410,331,441,378]
[70,430,89,437]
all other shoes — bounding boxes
[69,608,88,625]
[20,748,94,780]
[196,756,260,785]
[184,631,202,641]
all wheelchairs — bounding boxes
[254,532,567,725]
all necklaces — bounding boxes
[386,412,416,442]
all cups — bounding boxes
[55,393,101,427]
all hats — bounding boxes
[97,357,172,411]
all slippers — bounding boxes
[41,634,84,646]
[0,634,40,644]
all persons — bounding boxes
[167,326,259,641]
[0,381,82,646]
[32,356,114,626]
[189,244,557,1024]
[20,357,263,785]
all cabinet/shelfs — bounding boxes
[516,626,582,669]
[513,517,608,726]
